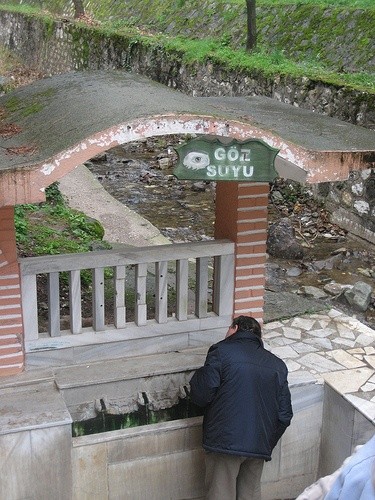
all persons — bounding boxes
[189,316,293,500]
